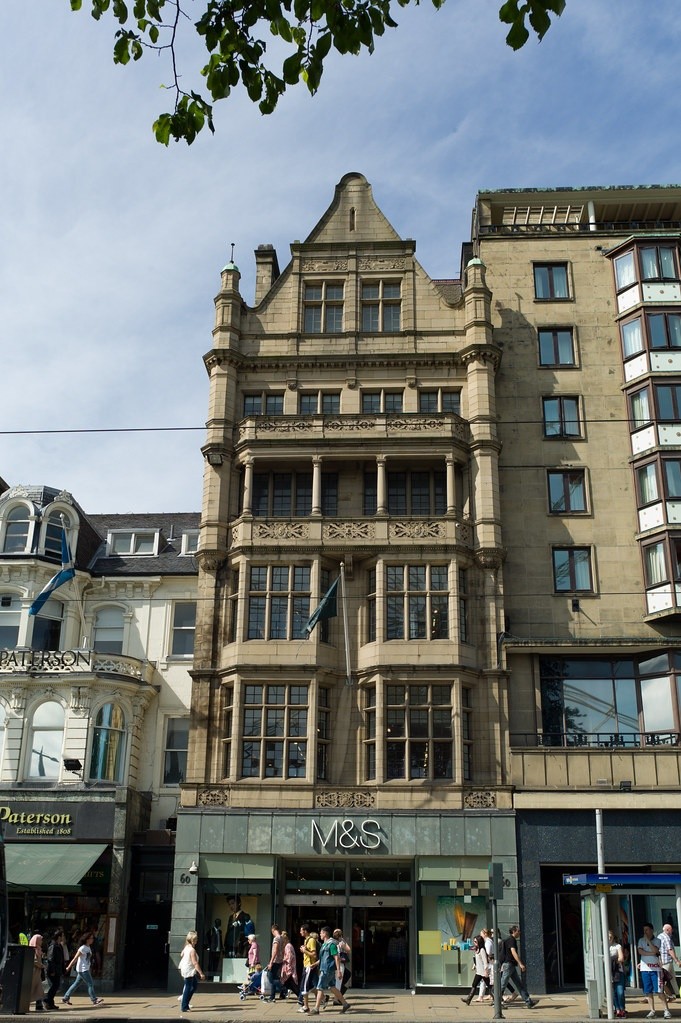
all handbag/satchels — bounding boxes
[339,952,351,962]
[472,954,489,965]
[661,967,676,1003]
[260,970,270,994]
[245,958,249,968]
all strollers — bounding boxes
[237,966,268,1001]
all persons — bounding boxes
[177,931,205,1012]
[637,923,681,1019]
[237,934,262,995]
[607,929,627,1019]
[29,934,48,1011]
[230,913,255,958]
[206,919,224,980]
[263,924,351,1016]
[461,925,540,1010]
[224,895,251,958]
[42,930,104,1011]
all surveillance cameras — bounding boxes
[188,866,198,874]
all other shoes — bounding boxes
[460,995,472,1006]
[263,995,351,1016]
[177,995,192,1008]
[640,998,648,1003]
[647,1009,672,1019]
[526,1000,539,1009]
[61,998,73,1005]
[36,1005,59,1011]
[471,993,518,1009]
[614,1010,627,1018]
[93,998,104,1007]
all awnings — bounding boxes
[3,843,109,894]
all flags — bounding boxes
[28,528,76,618]
[299,576,340,636]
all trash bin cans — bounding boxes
[3,945,35,1015]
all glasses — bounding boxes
[474,940,477,943]
[490,932,493,933]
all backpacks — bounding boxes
[306,937,322,962]
[498,936,513,963]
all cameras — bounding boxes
[41,958,48,965]
